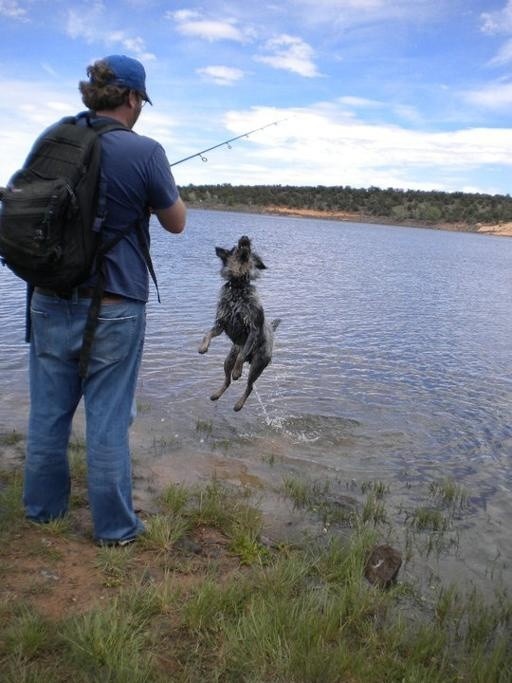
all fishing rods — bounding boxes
[169,118,294,167]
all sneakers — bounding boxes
[95,519,147,546]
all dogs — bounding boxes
[198,234,282,413]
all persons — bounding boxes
[0,54,186,548]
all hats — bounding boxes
[89,55,152,105]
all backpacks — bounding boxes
[0,117,133,301]
